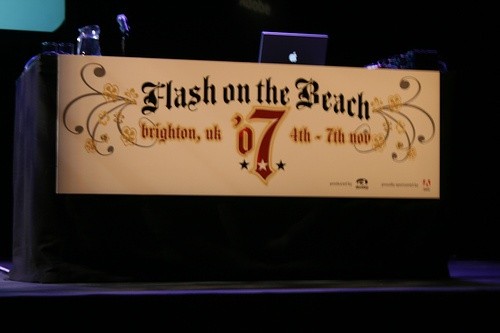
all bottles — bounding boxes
[76,25,102,54]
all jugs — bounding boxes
[27,42,75,71]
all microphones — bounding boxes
[117,14,129,33]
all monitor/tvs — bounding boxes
[260,31,328,65]
